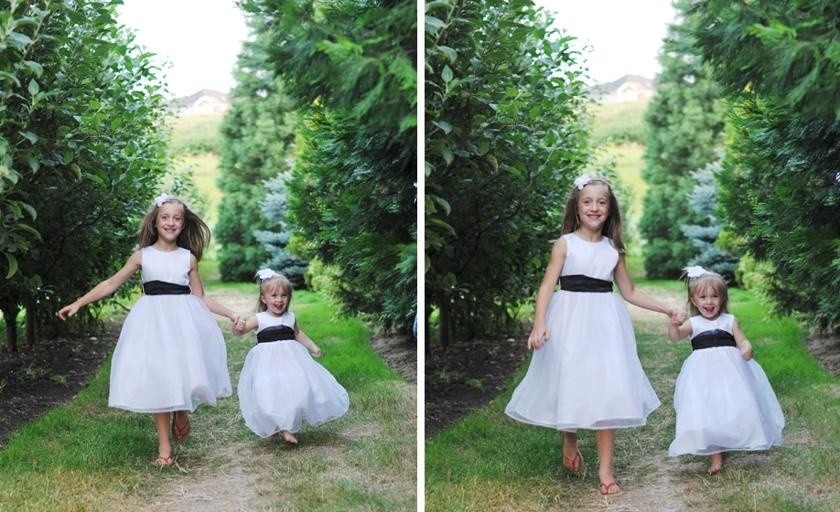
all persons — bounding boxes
[669,266,785,475]
[235,268,350,443]
[59,196,246,470]
[503,173,688,500]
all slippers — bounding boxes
[172,411,190,439]
[562,445,586,474]
[156,454,175,467]
[599,478,623,499]
